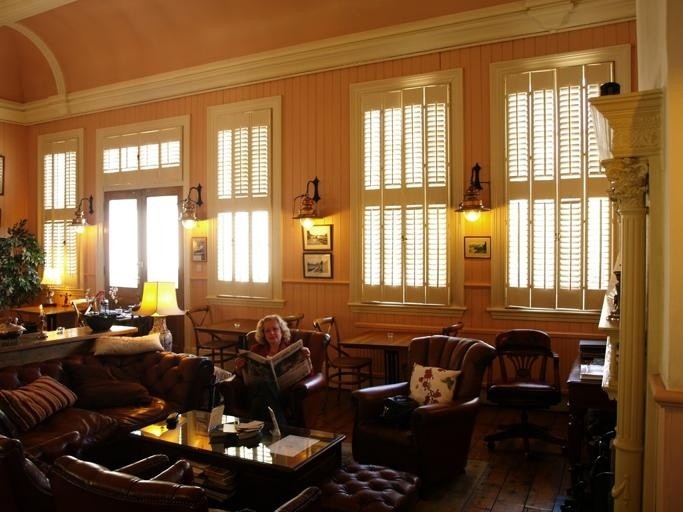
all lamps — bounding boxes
[129,282,186,352]
[68,190,96,226]
[179,181,205,230]
[288,177,327,231]
[457,159,491,224]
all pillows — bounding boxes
[94,336,163,354]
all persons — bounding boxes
[235,314,313,428]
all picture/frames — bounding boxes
[301,256,335,280]
[189,236,207,261]
[300,224,332,251]
[463,234,493,260]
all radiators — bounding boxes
[353,320,609,398]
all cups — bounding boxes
[600,81,620,95]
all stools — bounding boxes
[325,460,422,511]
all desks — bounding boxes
[0,297,143,337]
[338,332,434,384]
[190,318,270,363]
[569,339,615,508]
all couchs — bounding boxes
[220,331,332,429]
[1,346,320,512]
[351,336,497,486]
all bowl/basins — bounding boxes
[0,329,23,340]
[80,313,116,331]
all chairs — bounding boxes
[443,319,464,338]
[314,318,372,415]
[187,304,241,370]
[485,330,566,460]
[284,314,306,329]
